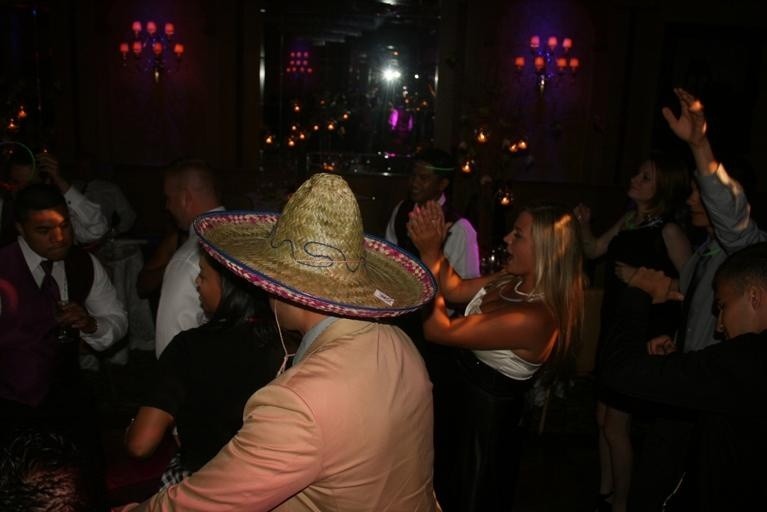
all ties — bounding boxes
[36,258,63,308]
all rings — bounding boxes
[576,216,581,220]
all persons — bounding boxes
[381,148,481,511]
[2,182,129,420]
[113,170,442,510]
[155,156,225,360]
[405,198,586,510]
[124,247,298,487]
[2,429,82,510]
[3,138,136,277]
[648,82,766,512]
[587,240,767,511]
[570,154,692,511]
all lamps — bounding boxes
[285,48,315,81]
[514,35,580,102]
[117,20,186,84]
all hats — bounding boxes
[190,166,442,323]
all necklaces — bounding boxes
[623,207,662,232]
[497,281,534,306]
[512,279,543,298]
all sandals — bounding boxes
[595,489,615,512]
